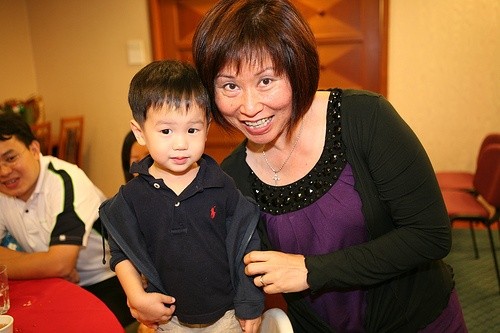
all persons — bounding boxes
[98,59,266,333]
[0,110,138,329]
[138,0,467,333]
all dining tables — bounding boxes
[0,277,126,333]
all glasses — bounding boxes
[1,147,28,166]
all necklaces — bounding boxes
[257,116,303,186]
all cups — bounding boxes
[0,264,10,315]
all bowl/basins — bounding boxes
[0,315,13,333]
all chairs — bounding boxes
[434,133,500,284]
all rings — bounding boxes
[261,275,265,286]
